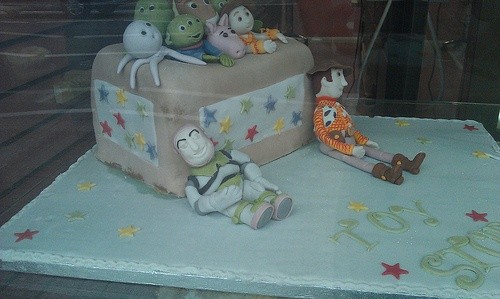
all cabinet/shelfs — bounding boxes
[90,41,316,197]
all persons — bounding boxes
[306,54,426,185]
[167,114,295,232]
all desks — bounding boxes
[0,115,500,299]
